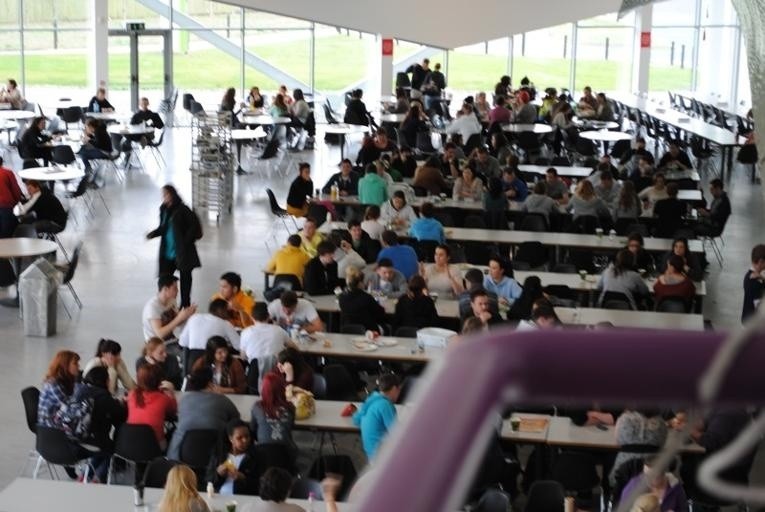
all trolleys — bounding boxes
[186,108,237,223]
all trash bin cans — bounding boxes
[18,257,60,337]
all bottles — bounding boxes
[307,491,316,511]
[207,482,214,497]
[564,497,575,511]
[326,210,332,225]
[385,211,411,236]
[330,184,340,201]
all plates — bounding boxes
[348,336,399,352]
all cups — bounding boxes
[509,417,521,434]
[579,222,649,284]
[133,485,144,506]
[421,188,447,208]
[291,324,308,344]
[337,259,465,310]
[225,502,236,512]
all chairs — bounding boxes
[147,235,764,375]
[0,352,765,512]
[1,90,167,225]
[1,226,84,319]
[184,90,765,239]
